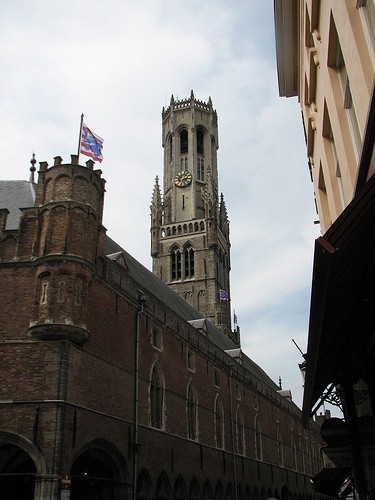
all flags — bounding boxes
[79,122,105,164]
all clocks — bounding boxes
[174,171,193,188]
